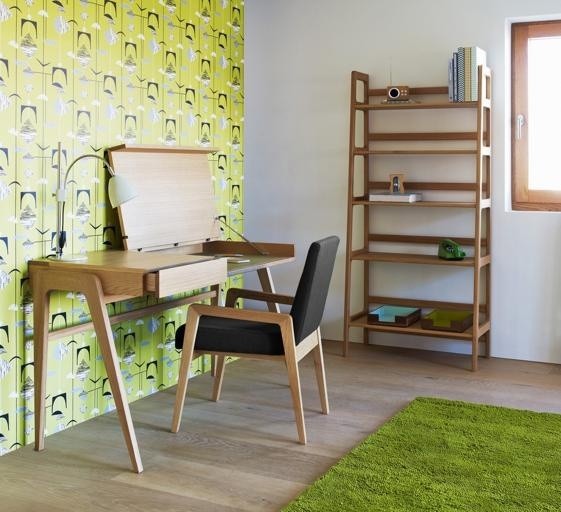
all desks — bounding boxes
[27,145,295,474]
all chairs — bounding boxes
[170,235,341,444]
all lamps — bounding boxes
[47,142,139,262]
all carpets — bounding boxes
[278,397,561,512]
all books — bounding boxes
[368,192,423,203]
[448,45,486,103]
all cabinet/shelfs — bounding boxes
[342,64,492,372]
